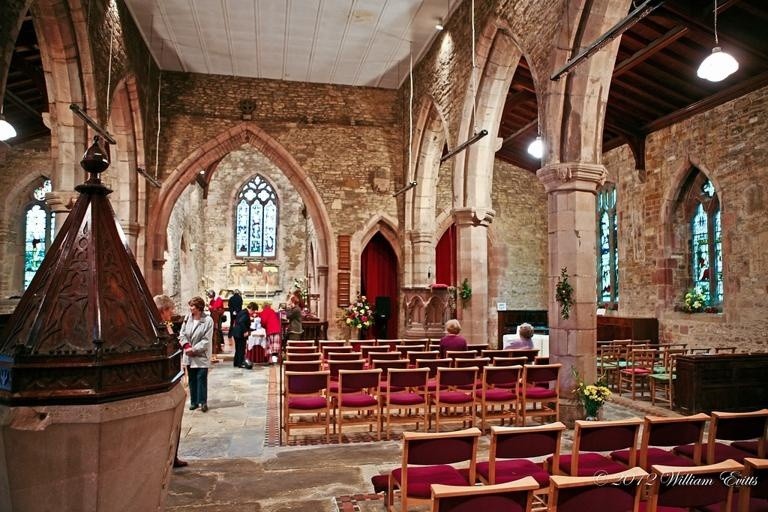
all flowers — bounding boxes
[686,288,707,309]
[458,277,472,303]
[555,264,576,321]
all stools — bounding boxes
[287,330,304,341]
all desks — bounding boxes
[244,327,268,363]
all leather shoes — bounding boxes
[189,403,198,410]
[174,456,187,468]
[202,403,207,412]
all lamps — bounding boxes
[69,0,117,145]
[435,17,443,31]
[696,0,739,82]
[439,0,489,163]
[550,0,667,82]
[528,135,547,159]
[137,16,165,189]
[392,40,418,197]
[0,107,18,142]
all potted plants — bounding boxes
[691,306,704,313]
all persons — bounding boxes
[153,294,188,467]
[504,323,534,350]
[285,296,304,340]
[260,302,282,365]
[178,296,214,412]
[439,318,468,359]
[206,289,224,354]
[228,288,242,338]
[231,302,258,368]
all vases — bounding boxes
[342,295,375,330]
